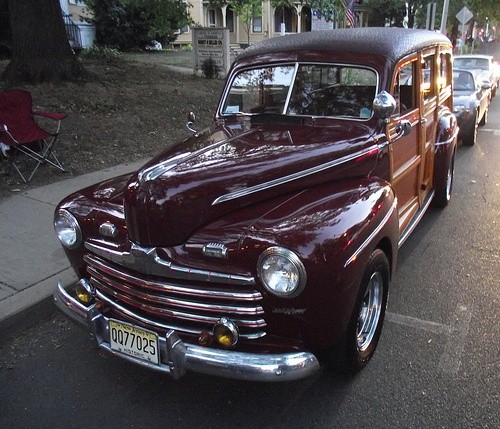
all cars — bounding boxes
[49,27,459,382]
[404,68,492,146]
[453,54,499,98]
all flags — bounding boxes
[340,0,360,28]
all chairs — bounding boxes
[292,85,373,112]
[0,90,68,184]
